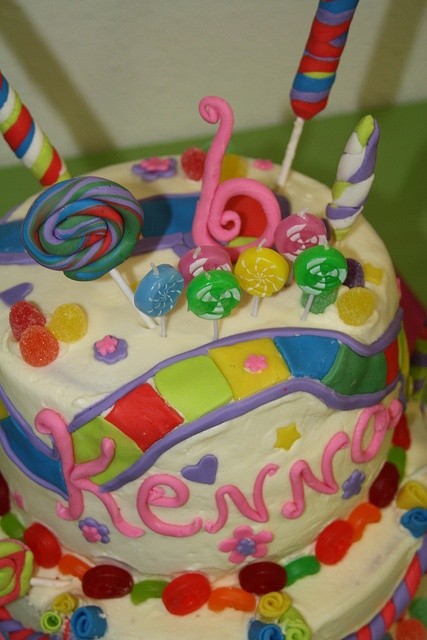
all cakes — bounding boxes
[1,0,425,640]
